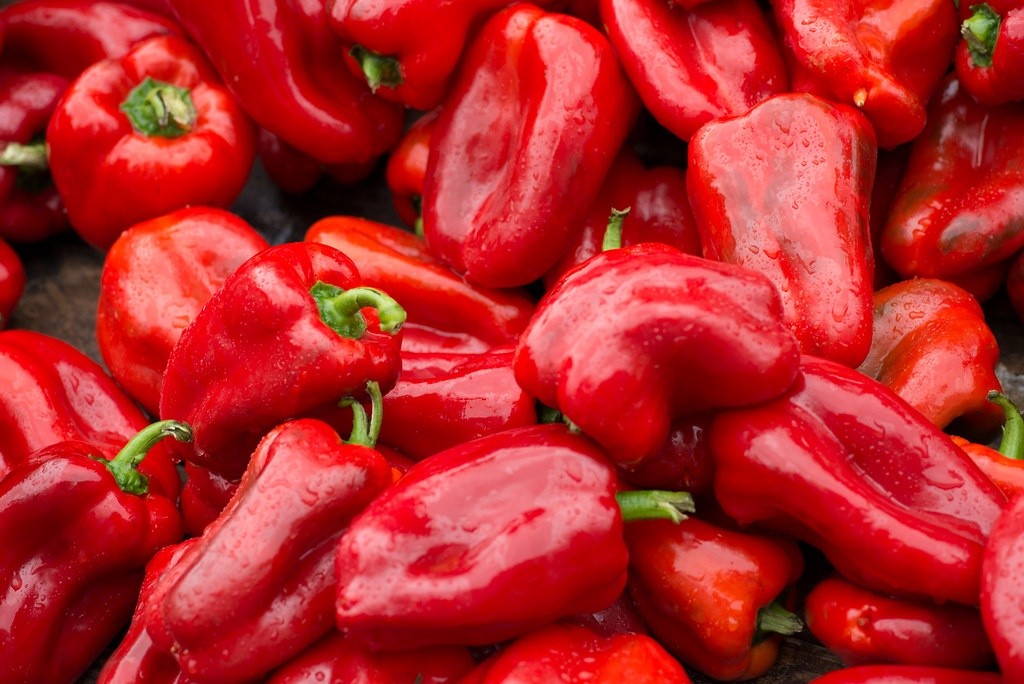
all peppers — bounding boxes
[0,0,1023,684]
